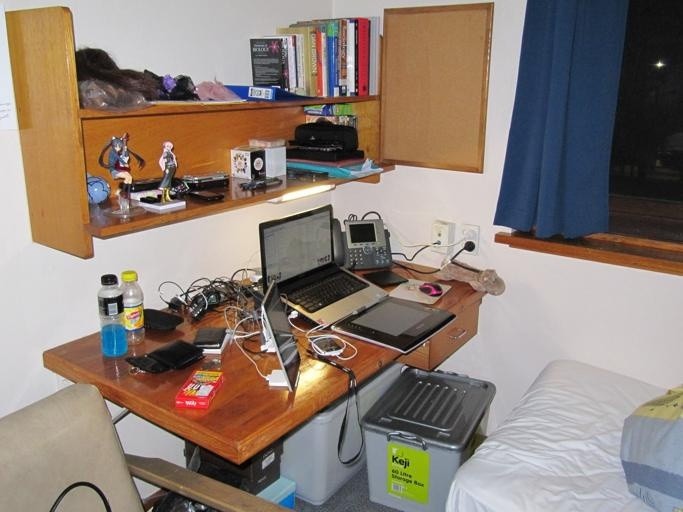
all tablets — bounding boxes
[260,281,303,394]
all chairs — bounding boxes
[0,383,297,512]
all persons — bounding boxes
[156,140,178,206]
[98,131,136,200]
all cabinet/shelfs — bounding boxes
[5,6,396,261]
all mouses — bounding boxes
[419,280,443,297]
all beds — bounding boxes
[445,359,683,512]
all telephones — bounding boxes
[332,218,392,270]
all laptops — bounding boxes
[258,204,390,329]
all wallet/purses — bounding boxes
[148,340,206,369]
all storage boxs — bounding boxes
[279,363,404,505]
[360,367,495,512]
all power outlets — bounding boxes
[456,224,479,254]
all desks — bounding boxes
[42,261,486,466]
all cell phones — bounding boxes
[309,334,342,356]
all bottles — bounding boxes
[97,273,128,359]
[119,270,145,346]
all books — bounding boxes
[250,15,379,98]
[287,105,367,172]
